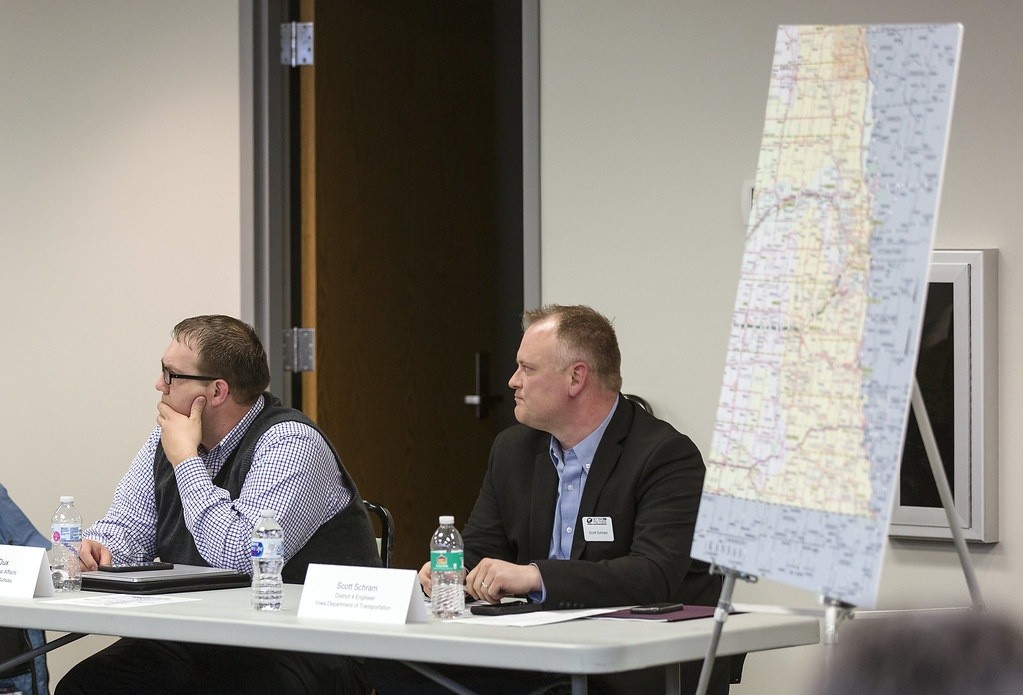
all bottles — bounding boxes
[51,497,82,590]
[250,508,282,613]
[429,515,466,622]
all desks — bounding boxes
[0,582,821,695]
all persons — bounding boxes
[52,314,383,695]
[364,305,724,695]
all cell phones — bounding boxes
[95,560,174,572]
[631,602,684,614]
[474,598,544,615]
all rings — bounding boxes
[482,582,489,588]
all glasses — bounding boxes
[161,358,230,396]
